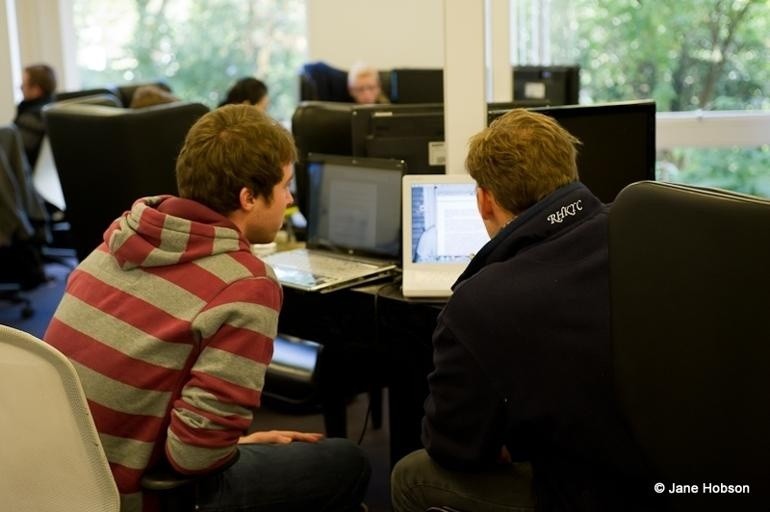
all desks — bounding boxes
[252,230,454,474]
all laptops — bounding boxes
[257,152,408,292]
[401,176,493,298]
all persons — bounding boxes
[396,108,668,511]
[39,101,371,512]
[217,78,268,107]
[347,61,397,105]
[132,84,183,108]
[13,66,56,173]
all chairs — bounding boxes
[0,323,119,512]
[608,179,770,512]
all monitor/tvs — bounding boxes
[352,102,448,174]
[488,100,657,202]
[511,64,580,106]
[489,102,525,110]
[120,85,171,112]
[388,67,443,102]
[54,88,117,100]
